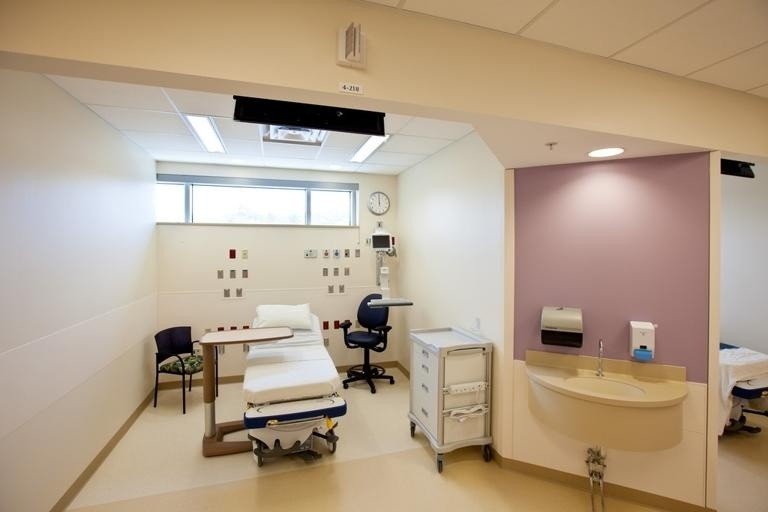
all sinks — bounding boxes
[564,376,646,399]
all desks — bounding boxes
[200,326,295,458]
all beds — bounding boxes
[242,298,350,467]
[720,341,768,437]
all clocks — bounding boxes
[366,191,390,215]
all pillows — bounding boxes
[254,304,313,330]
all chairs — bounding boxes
[340,293,396,393]
[152,325,220,416]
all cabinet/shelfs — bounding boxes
[406,325,492,452]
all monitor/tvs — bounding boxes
[372,234,392,252]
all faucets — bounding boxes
[597,339,604,376]
